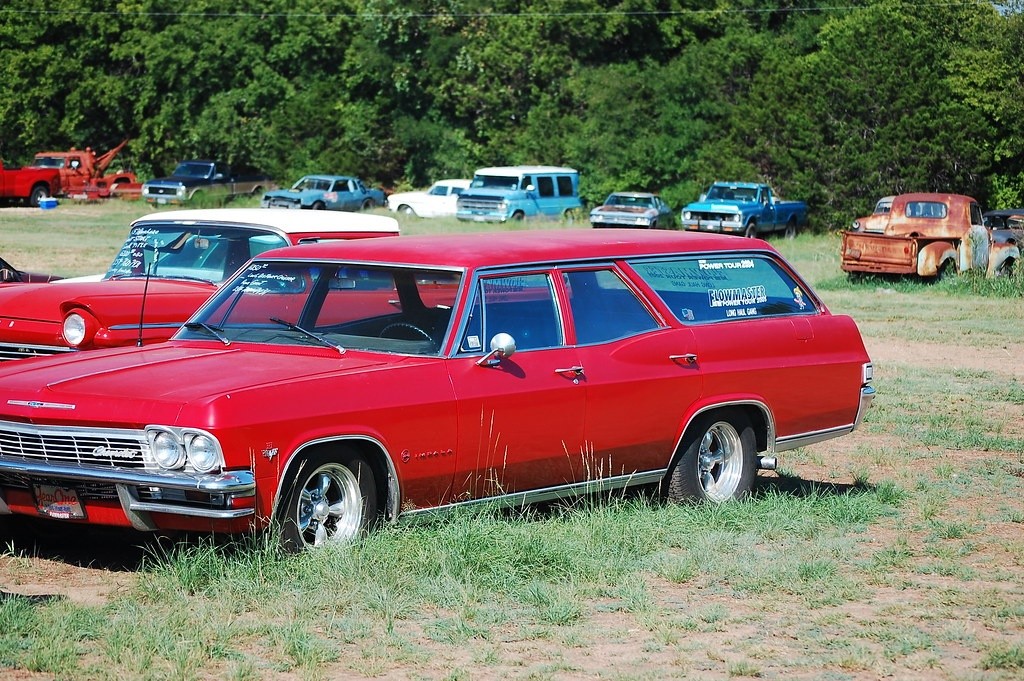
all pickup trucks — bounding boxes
[680,180,812,243]
[141,159,278,209]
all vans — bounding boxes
[455,164,583,225]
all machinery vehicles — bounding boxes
[31,129,137,201]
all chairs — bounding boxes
[727,192,734,200]
[754,192,764,203]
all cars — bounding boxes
[0,229,874,559]
[840,192,1024,285]
[0,155,64,206]
[0,206,400,366]
[589,191,675,231]
[261,175,385,212]
[388,178,472,221]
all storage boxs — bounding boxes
[40,198,56,210]
